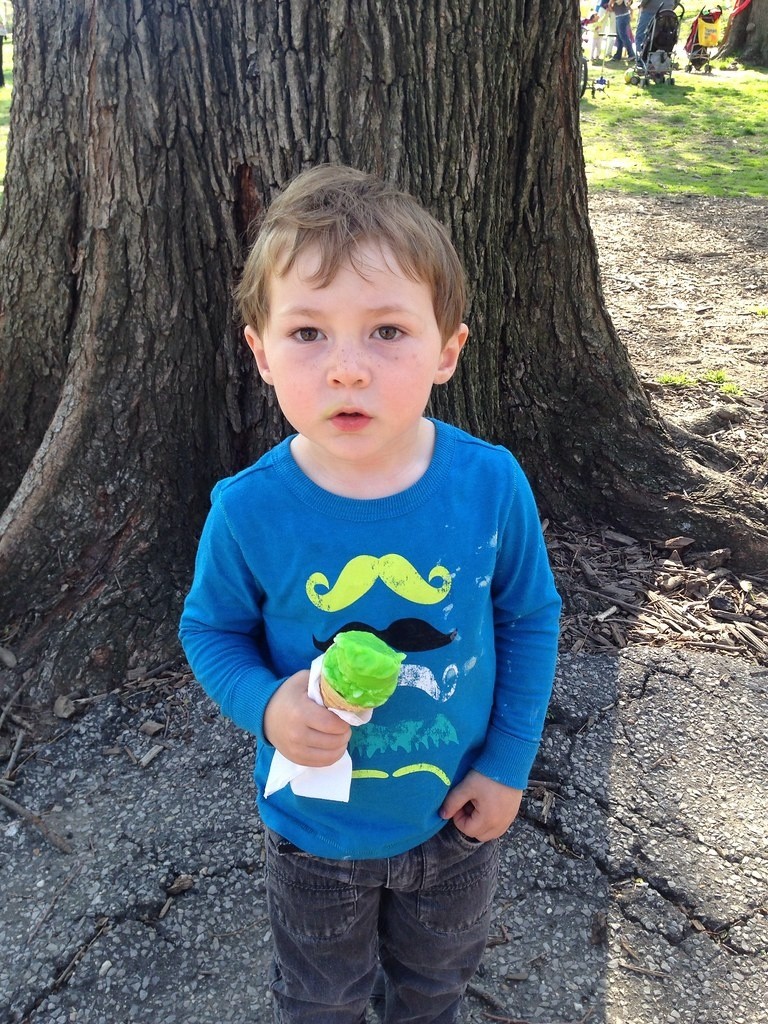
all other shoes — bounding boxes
[590,58,594,60]
[609,57,621,61]
[595,57,601,60]
[626,55,636,62]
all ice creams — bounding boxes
[319,630,407,713]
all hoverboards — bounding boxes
[593,32,618,92]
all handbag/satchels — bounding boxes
[697,16,720,46]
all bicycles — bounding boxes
[580,27,589,100]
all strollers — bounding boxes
[681,5,723,75]
[628,0,684,90]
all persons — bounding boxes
[176,164,562,1024]
[588,0,681,62]
